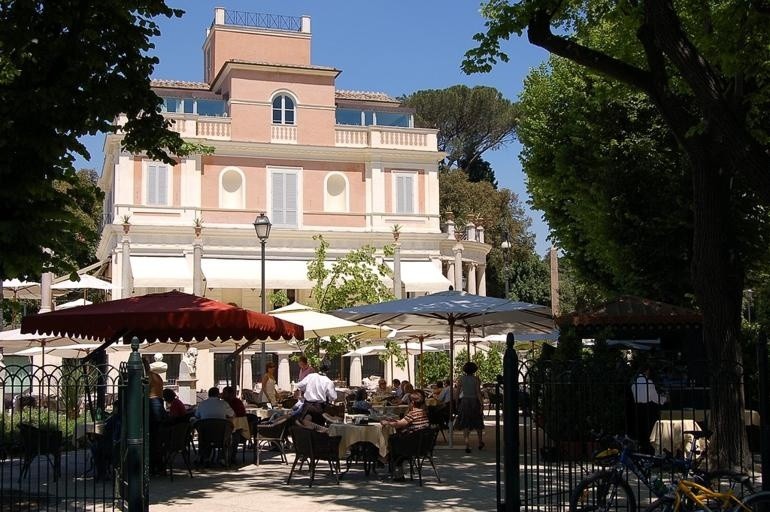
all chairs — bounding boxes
[73,383,503,488]
[17,423,62,483]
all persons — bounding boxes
[628,365,667,449]
[163,389,186,416]
[291,357,315,411]
[194,388,235,465]
[381,389,430,478]
[353,388,372,411]
[375,378,414,406]
[142,356,165,419]
[433,379,450,403]
[296,359,337,461]
[262,363,279,404]
[454,361,484,453]
[219,387,246,418]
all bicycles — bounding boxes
[646,447,769,512]
[569,424,760,512]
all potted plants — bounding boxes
[529,330,646,462]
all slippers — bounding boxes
[376,456,386,468]
[391,476,405,482]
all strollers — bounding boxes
[257,400,306,453]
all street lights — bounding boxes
[500,225,512,298]
[253,211,272,315]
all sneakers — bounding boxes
[479,442,485,449]
[464,447,471,454]
